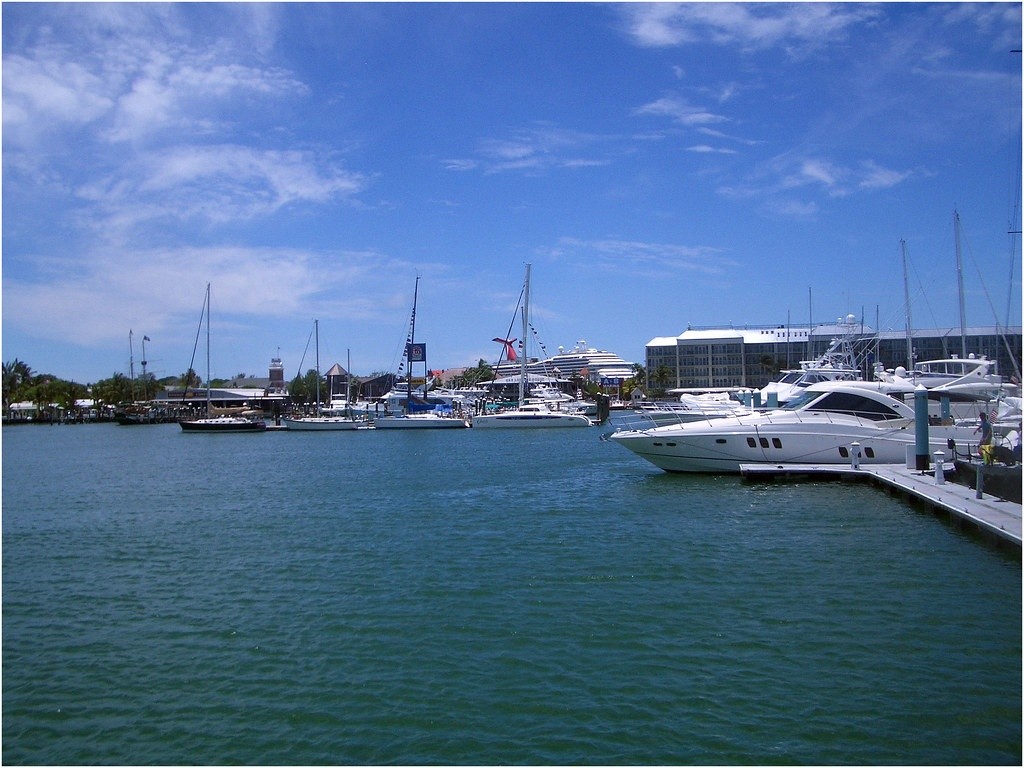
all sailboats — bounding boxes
[861,207,1022,419]
[177,281,266,433]
[279,320,370,431]
[472,259,597,428]
[115,329,165,424]
[372,276,472,430]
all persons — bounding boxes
[1014,422,1022,465]
[974,412,992,458]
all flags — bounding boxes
[990,410,998,424]
[143,336,150,341]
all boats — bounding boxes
[955,459,1022,505]
[599,286,1022,474]
